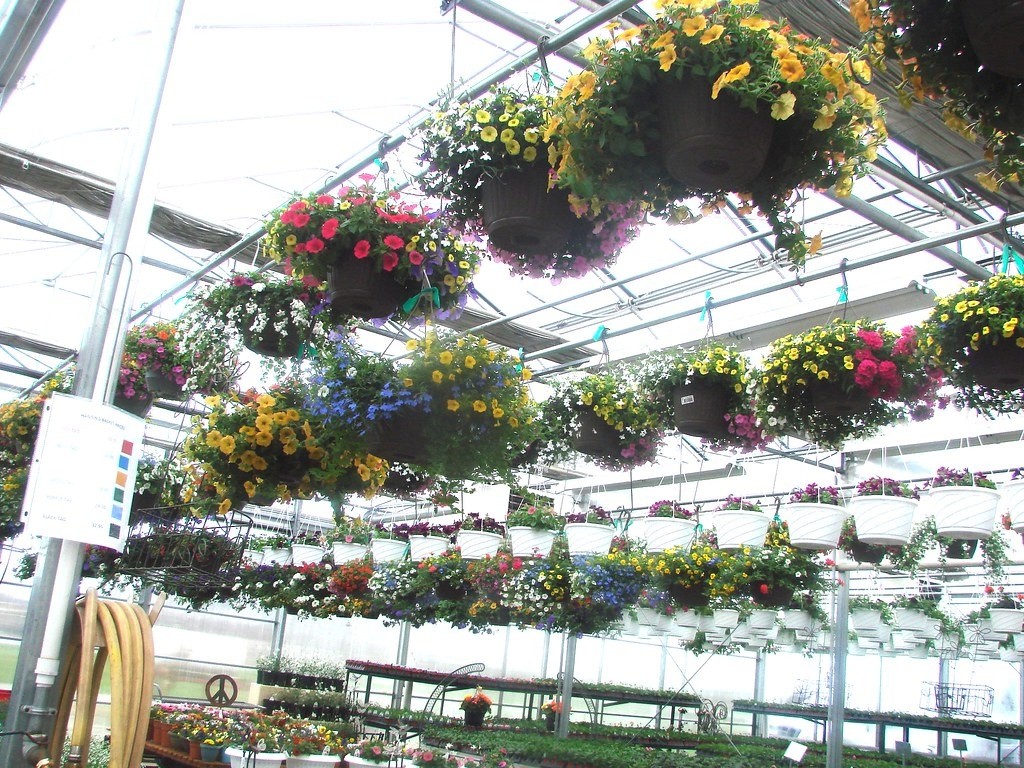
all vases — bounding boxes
[148,475,1024,768]
[671,382,729,439]
[112,385,153,418]
[812,378,866,414]
[660,81,772,192]
[323,259,407,319]
[143,369,184,395]
[963,0,1024,81]
[971,343,1023,387]
[571,410,626,456]
[237,308,301,355]
[133,492,154,508]
[479,164,576,254]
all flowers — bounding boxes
[925,467,998,489]
[788,484,844,508]
[754,317,951,454]
[839,513,907,567]
[894,274,1024,414]
[637,536,749,598]
[734,519,847,611]
[848,596,893,625]
[715,495,764,513]
[1,0,640,768]
[558,0,888,280]
[647,500,692,520]
[856,479,905,498]
[983,586,1024,610]
[889,594,946,622]
[847,0,1024,192]
[629,342,774,457]
[907,519,1014,587]
